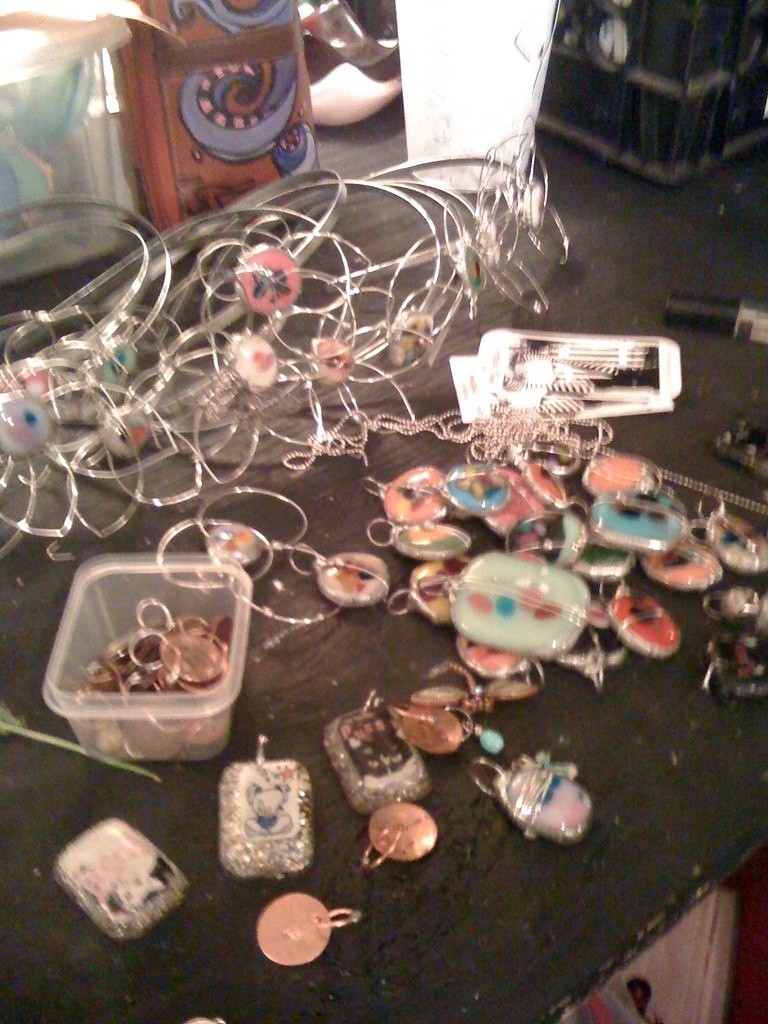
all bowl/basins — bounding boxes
[42,552,253,760]
